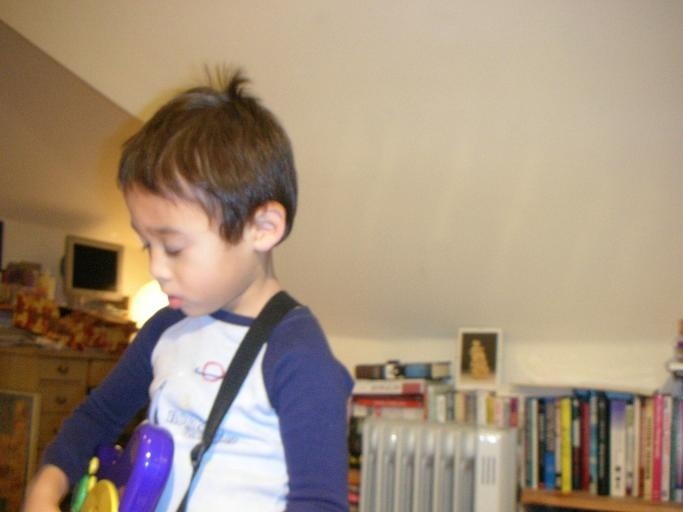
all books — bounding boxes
[349,360,452,469]
[426,383,518,426]
[518,387,682,500]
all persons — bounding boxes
[24,66,353,511]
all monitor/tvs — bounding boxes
[59,234,126,303]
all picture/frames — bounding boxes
[0,391,40,512]
[455,328,502,391]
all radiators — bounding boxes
[358,421,518,512]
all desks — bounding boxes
[0,347,147,469]
